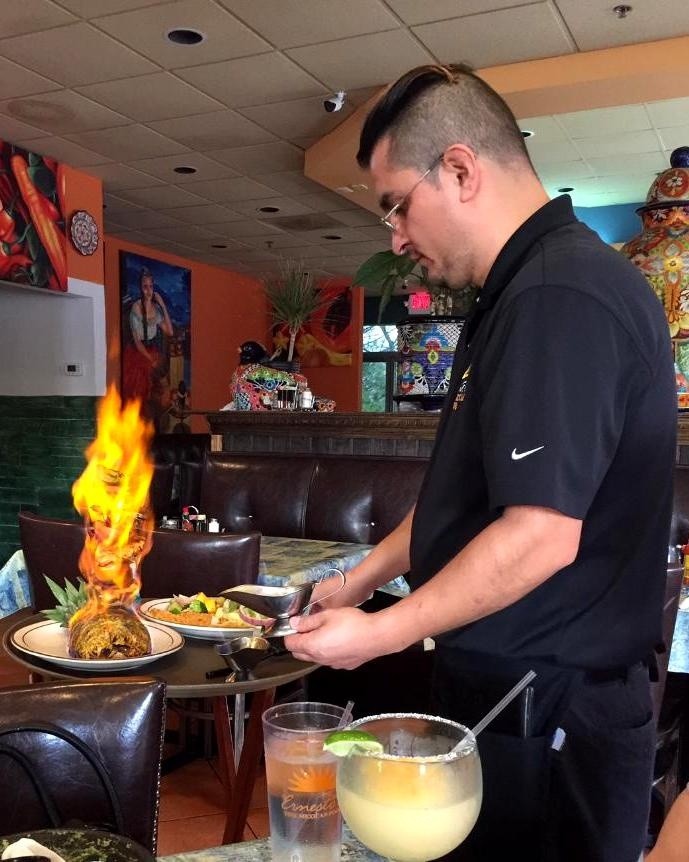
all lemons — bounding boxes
[323,730,383,757]
[168,598,259,617]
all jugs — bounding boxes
[217,567,346,638]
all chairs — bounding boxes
[149,431,213,523]
[17,509,262,612]
[0,676,168,862]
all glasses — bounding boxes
[376,154,444,232]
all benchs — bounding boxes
[199,453,689,574]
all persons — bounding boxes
[285,61,677,861]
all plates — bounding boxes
[10,618,185,672]
[138,597,260,641]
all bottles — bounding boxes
[159,506,221,533]
[301,388,313,408]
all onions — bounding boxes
[239,606,277,626]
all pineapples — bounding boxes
[39,572,152,661]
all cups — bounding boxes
[261,701,353,862]
[335,712,484,861]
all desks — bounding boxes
[3,598,321,850]
[252,534,412,608]
